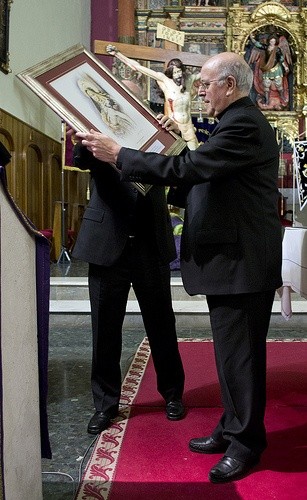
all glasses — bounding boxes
[201,79,226,89]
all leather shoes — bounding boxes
[166,399,185,421]
[208,455,258,483]
[189,436,231,453]
[87,411,119,435]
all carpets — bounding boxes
[74,336,307,500]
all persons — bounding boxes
[65,81,185,434]
[106,45,201,151]
[249,33,289,105]
[76,51,282,485]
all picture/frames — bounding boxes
[16,40,187,196]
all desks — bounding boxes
[276,226,307,322]
